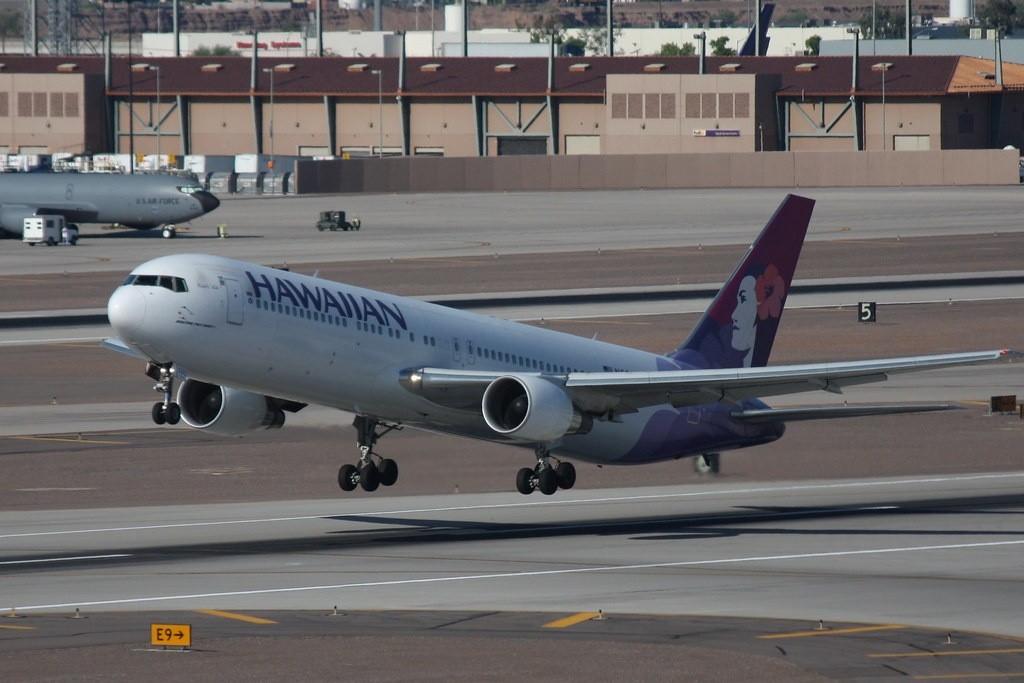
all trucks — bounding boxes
[22,215,78,245]
[317,210,360,231]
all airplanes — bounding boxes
[101,194,1024,493]
[0,172,220,239]
